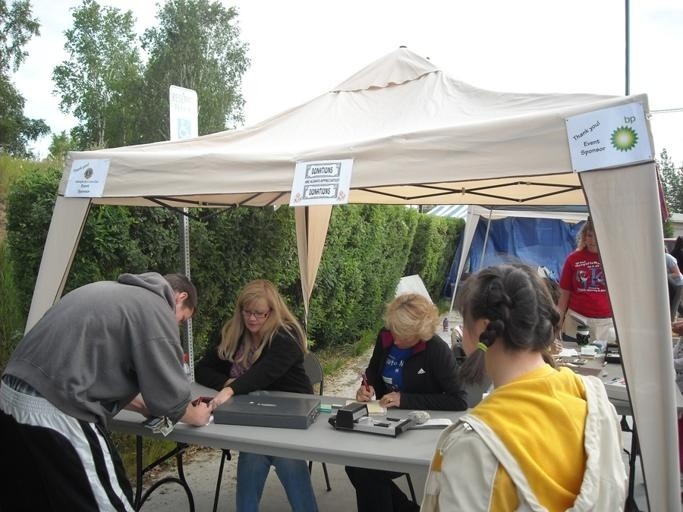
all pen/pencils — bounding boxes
[362,373,371,392]
[197,397,203,407]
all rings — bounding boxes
[387,397,392,402]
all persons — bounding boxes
[664,246,681,321]
[201,277,325,511]
[418,256,632,512]
[554,220,615,343]
[0,269,214,512]
[342,292,468,512]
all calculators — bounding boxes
[555,358,588,365]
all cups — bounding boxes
[576,325,591,352]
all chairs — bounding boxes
[302,351,332,489]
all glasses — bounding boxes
[241,309,271,318]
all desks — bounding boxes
[109,380,476,512]
